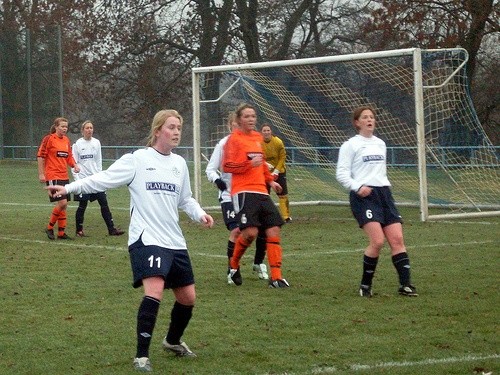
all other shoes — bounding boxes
[284,216,292,223]
[230,266,242,286]
[228,272,234,284]
[57,233,71,240]
[47,229,55,239]
[269,279,290,289]
[76,230,84,237]
[109,228,126,235]
[253,264,269,280]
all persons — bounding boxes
[36,117,81,240]
[260,124,292,223]
[220,102,290,288]
[70,120,126,238]
[336,107,420,297]
[205,111,271,284]
[42,110,214,373]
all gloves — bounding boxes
[215,179,227,191]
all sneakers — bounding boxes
[162,336,197,357]
[359,285,373,298]
[398,285,419,296]
[134,357,151,374]
